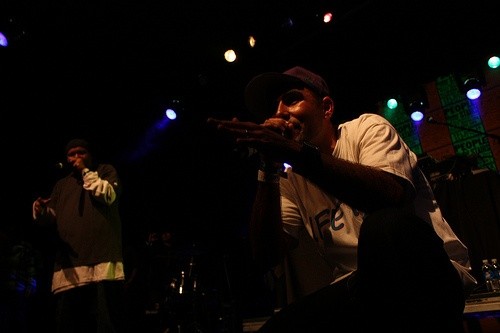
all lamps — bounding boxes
[410,100,424,123]
[464,79,482,100]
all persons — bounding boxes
[32,140,126,333]
[208,66,477,333]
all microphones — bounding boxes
[59,162,74,168]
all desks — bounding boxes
[463,292,500,314]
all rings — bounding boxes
[244,128,250,139]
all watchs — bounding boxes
[81,168,91,176]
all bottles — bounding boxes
[481,259,500,293]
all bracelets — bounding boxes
[292,141,321,179]
[257,169,287,183]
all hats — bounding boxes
[245,66,330,116]
